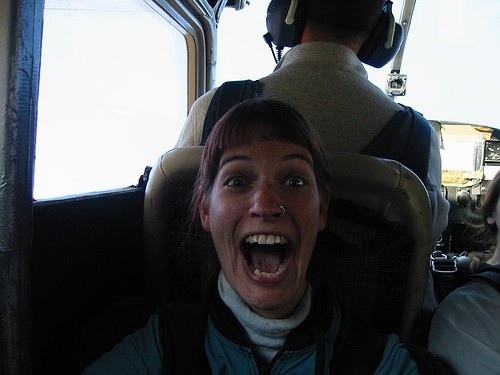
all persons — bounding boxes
[173,0,453,336]
[425,171,500,375]
[64,96,425,375]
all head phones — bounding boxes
[265,0,406,71]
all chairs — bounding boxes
[143,145,434,346]
